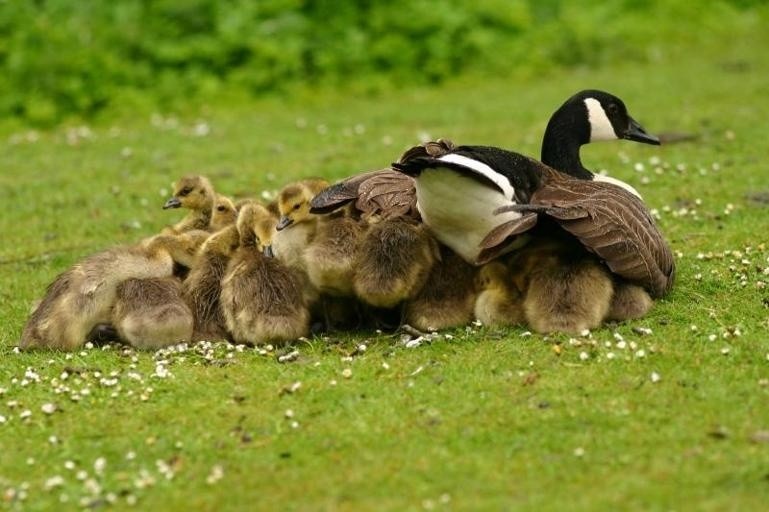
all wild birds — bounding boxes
[309,88,672,301]
[18,172,656,350]
